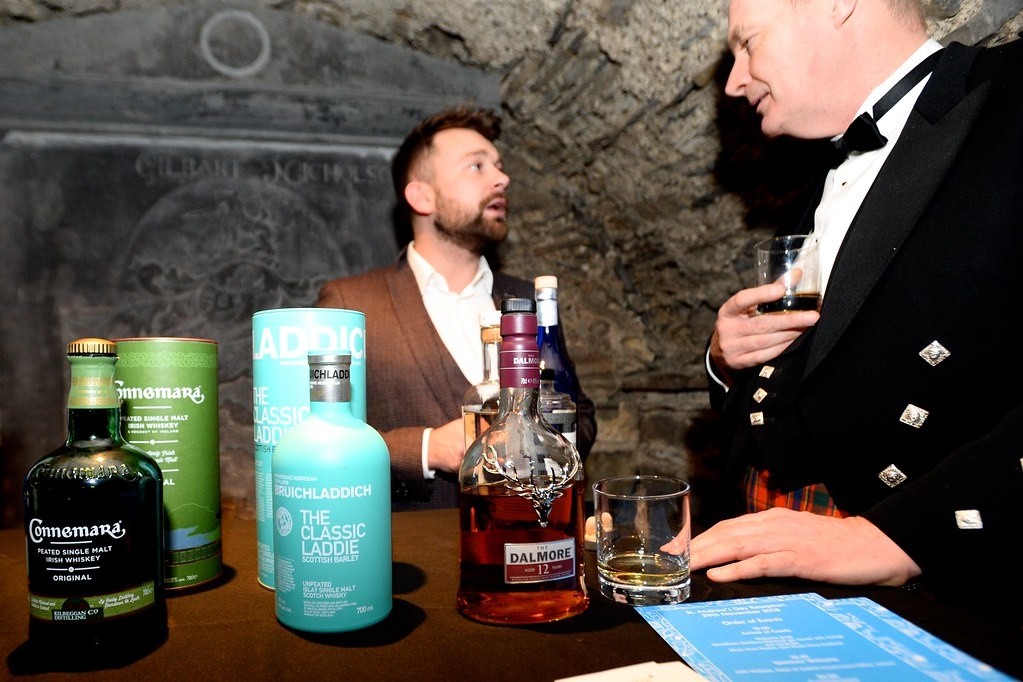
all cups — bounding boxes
[592,474,692,603]
[754,234,822,312]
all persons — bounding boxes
[313,106,597,512]
[689,0,1023,682]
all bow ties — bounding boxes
[811,47,947,168]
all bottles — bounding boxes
[22,339,167,667]
[272,352,394,634]
[457,275,587,626]
